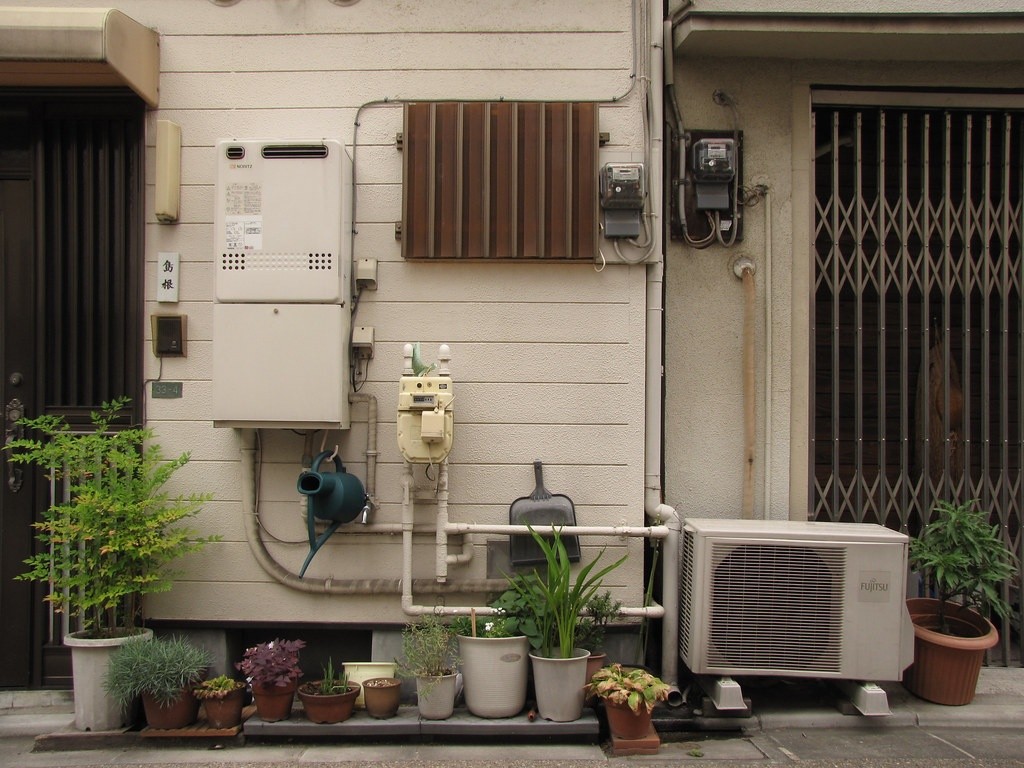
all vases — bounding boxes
[342,661,398,709]
[362,678,402,719]
[455,632,529,718]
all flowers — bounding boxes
[449,607,525,638]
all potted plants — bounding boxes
[568,591,622,708]
[0,395,225,731]
[101,632,216,729]
[582,662,670,740]
[192,674,246,729]
[901,499,1020,706]
[497,519,630,723]
[393,610,463,720]
[297,656,361,724]
[235,637,306,722]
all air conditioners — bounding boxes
[678,517,915,717]
